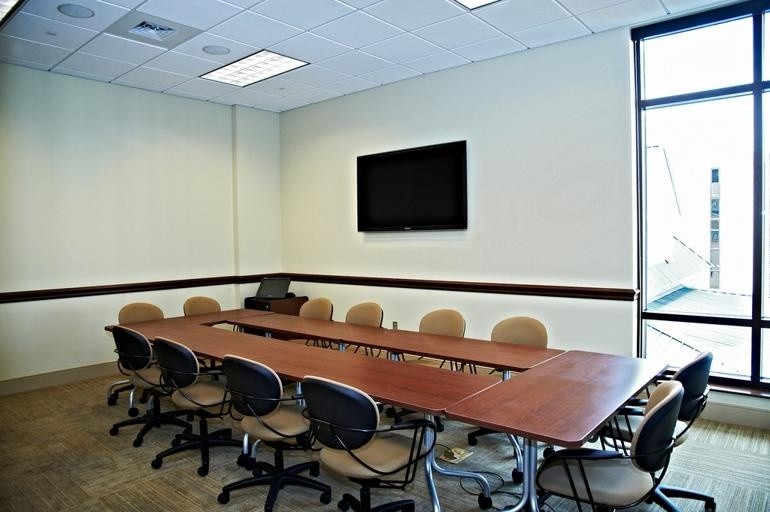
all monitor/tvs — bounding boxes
[255,276,291,299]
[356,140,469,233]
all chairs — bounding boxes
[345,301,384,359]
[299,374,438,511]
[489,316,548,381]
[183,296,221,316]
[107,302,164,417]
[414,309,466,372]
[108,325,195,447]
[299,297,334,348]
[533,379,716,512]
[217,352,332,512]
[600,350,713,449]
[151,336,250,477]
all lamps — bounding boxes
[454,0,501,11]
[199,49,311,89]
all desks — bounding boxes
[243,292,309,341]
[223,313,566,484]
[103,307,275,417]
[149,325,502,512]
[444,349,668,512]
